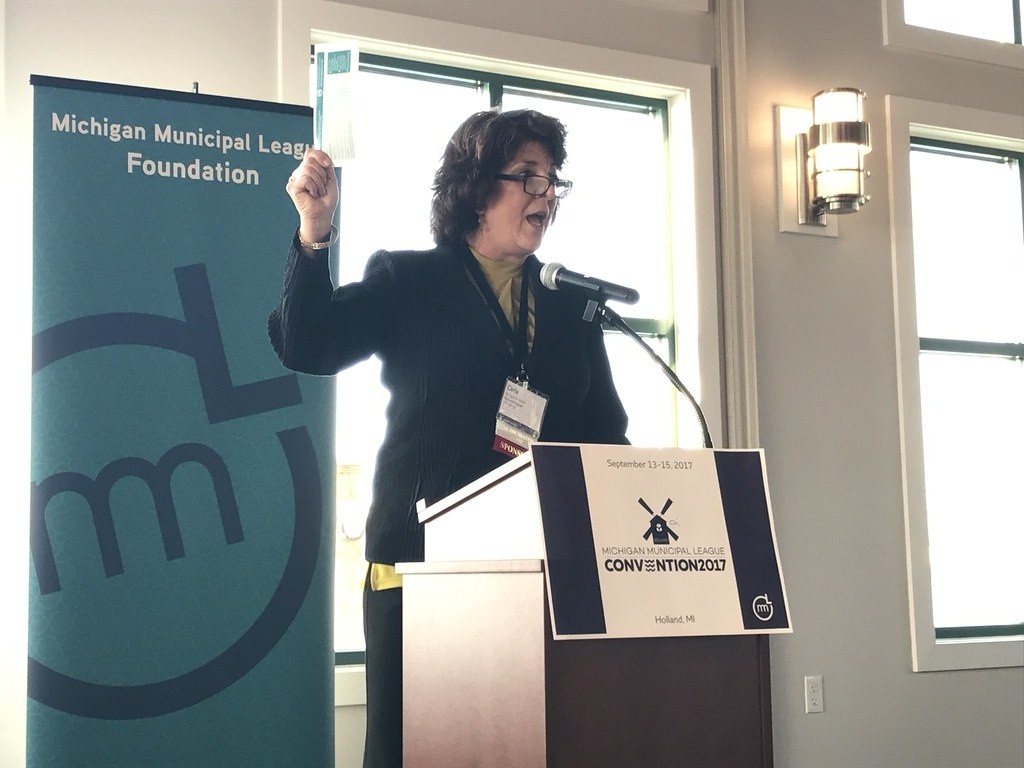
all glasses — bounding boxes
[495,173,574,198]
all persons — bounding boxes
[267,108,630,768]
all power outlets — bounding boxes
[803,674,824,714]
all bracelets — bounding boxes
[297,224,340,249]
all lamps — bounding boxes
[774,87,872,238]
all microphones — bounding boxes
[540,262,639,305]
